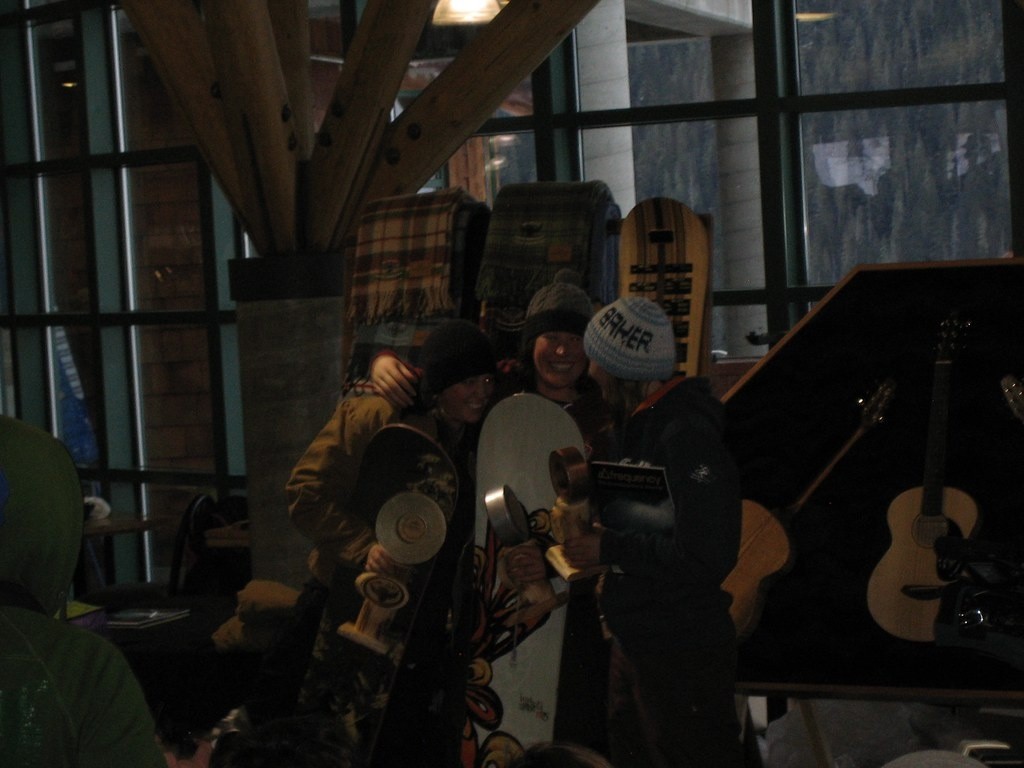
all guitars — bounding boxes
[865,311,980,644]
[720,371,904,646]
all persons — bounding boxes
[279,268,764,768]
[0,415,169,768]
[208,714,353,768]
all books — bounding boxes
[590,460,676,542]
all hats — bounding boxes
[423,320,496,393]
[522,268,594,345]
[583,296,675,381]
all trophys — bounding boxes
[551,447,613,584]
[336,491,447,654]
[484,483,570,629]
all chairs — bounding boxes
[77,494,246,757]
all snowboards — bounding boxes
[459,391,590,767]
[289,424,460,768]
